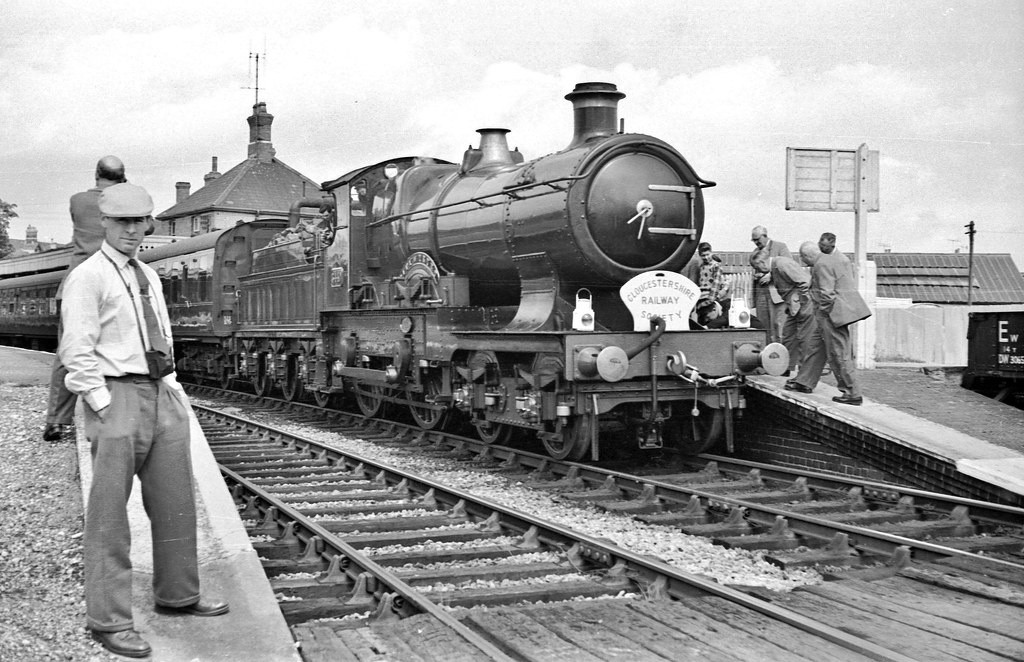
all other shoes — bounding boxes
[43,423,63,442]
[780,370,790,377]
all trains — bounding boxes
[0,52,792,466]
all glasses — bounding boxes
[752,234,763,241]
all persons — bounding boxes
[682,242,731,329]
[748,225,872,405]
[43,155,155,441]
[60,182,231,658]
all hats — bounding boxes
[98,182,154,217]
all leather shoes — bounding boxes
[833,394,862,405]
[154,596,229,616]
[91,628,152,658]
[785,379,812,393]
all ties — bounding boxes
[128,259,170,355]
